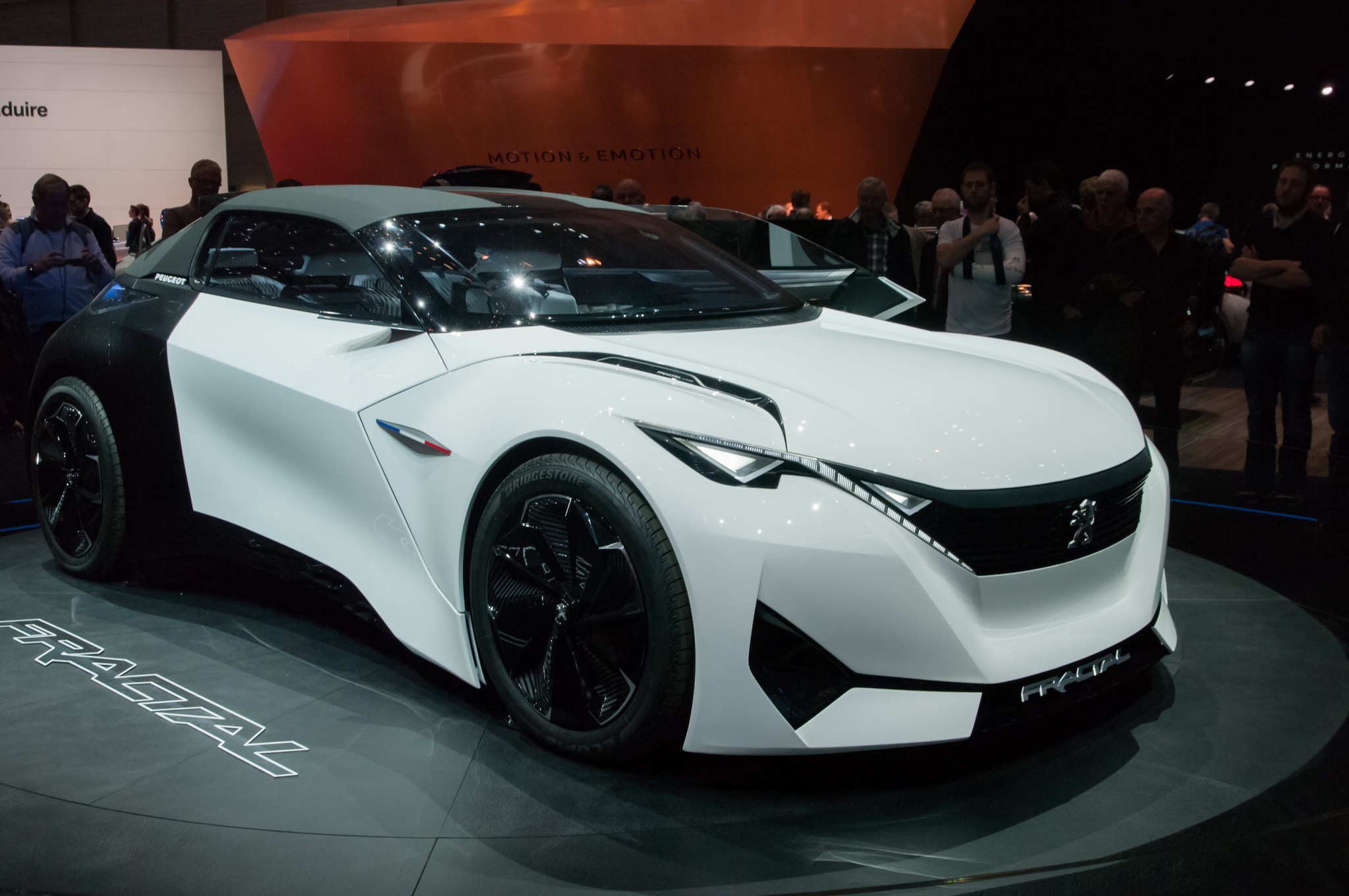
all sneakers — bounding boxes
[1236,481,1265,497]
[1274,477,1302,500]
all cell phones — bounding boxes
[60,258,83,266]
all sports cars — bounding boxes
[24,185,1177,776]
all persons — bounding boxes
[0,174,157,366]
[584,164,960,331]
[162,161,222,240]
[937,159,1349,496]
